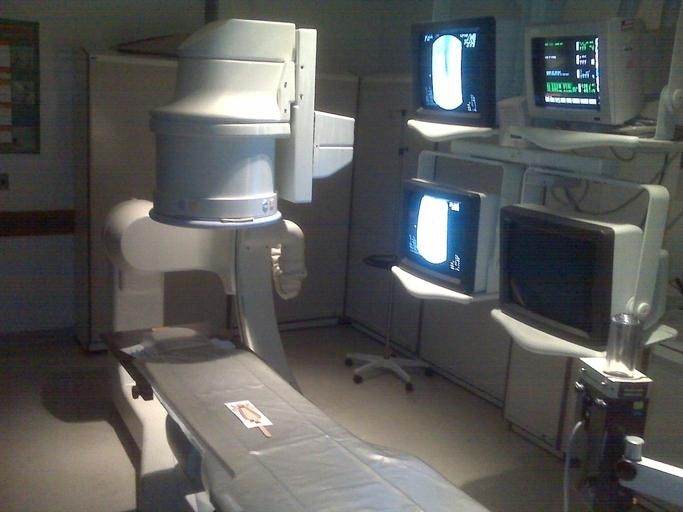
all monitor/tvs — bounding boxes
[499,202,643,352]
[524,15,645,128]
[397,178,500,294]
[410,15,524,128]
